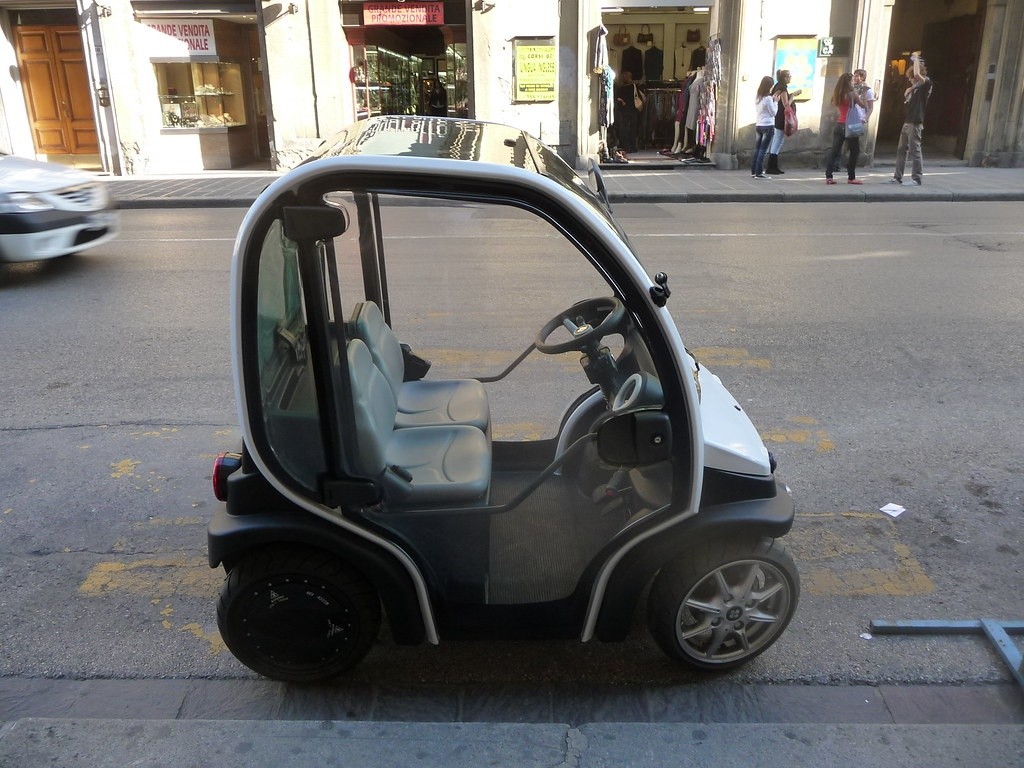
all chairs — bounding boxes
[332,302,492,507]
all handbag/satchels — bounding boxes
[846,93,864,138]
[633,83,643,111]
[614,26,630,46]
[687,28,700,41]
[638,25,653,43]
[784,102,798,137]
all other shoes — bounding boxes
[834,166,840,172]
[912,178,921,186]
[826,177,837,184]
[657,147,710,163]
[601,148,628,163]
[752,172,772,180]
[889,177,902,184]
[848,179,863,184]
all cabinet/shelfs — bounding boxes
[353,42,468,122]
[156,63,247,129]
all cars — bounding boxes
[0,150,120,263]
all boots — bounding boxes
[766,153,784,174]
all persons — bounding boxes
[888,52,933,184]
[615,72,646,153]
[765,69,802,174]
[670,72,697,153]
[750,76,780,179]
[832,70,874,172]
[825,73,862,185]
[429,79,446,116]
[681,70,704,153]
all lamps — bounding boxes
[693,7,710,15]
